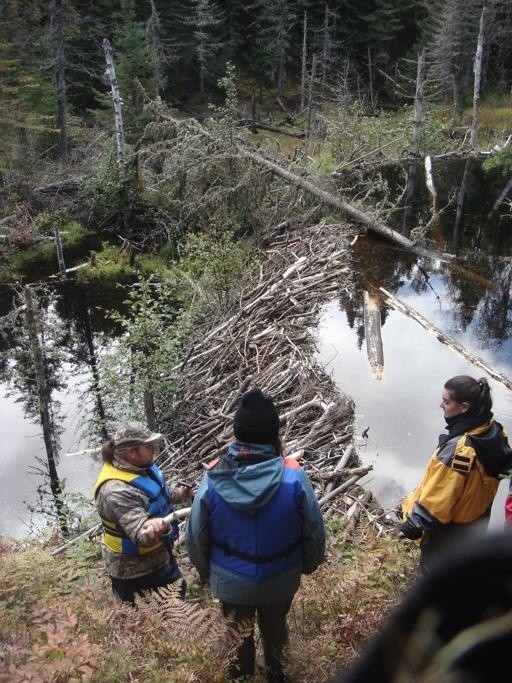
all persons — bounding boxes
[186,388,325,683]
[94,421,194,612]
[398,376,511,577]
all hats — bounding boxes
[112,420,164,449]
[230,386,282,442]
